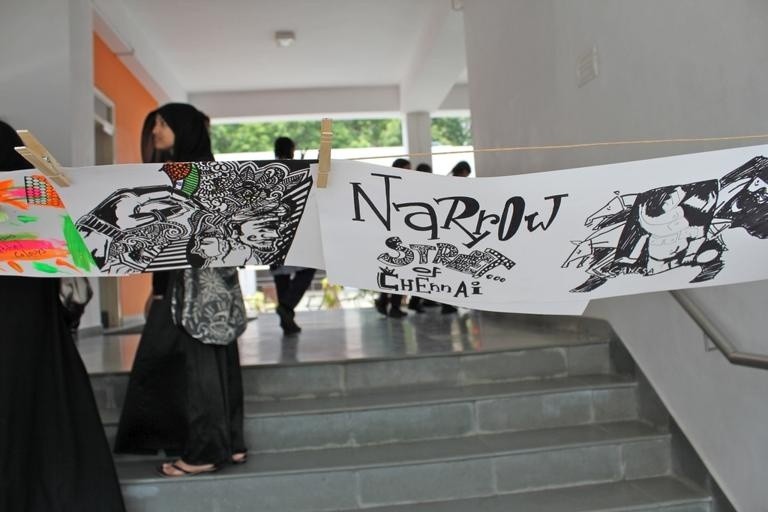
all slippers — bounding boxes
[158,453,217,475]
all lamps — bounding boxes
[278,26,297,48]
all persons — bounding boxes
[270,137,315,334]
[111,103,246,478]
[185,161,312,268]
[443,160,470,312]
[1,121,125,512]
[376,158,411,317]
[408,163,437,311]
